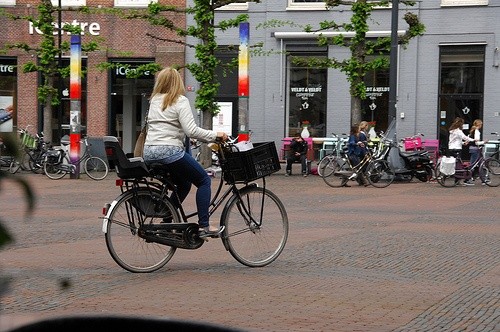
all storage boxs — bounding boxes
[405,137,422,149]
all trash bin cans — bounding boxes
[61,136,87,175]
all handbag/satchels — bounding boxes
[134,122,147,157]
[231,140,254,151]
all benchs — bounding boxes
[318,141,349,160]
[406,139,439,159]
[280,138,314,174]
[483,141,499,158]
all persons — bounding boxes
[441,117,492,186]
[142,66,229,243]
[285,134,309,177]
[339,121,382,187]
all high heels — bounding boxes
[198,225,225,242]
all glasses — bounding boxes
[294,137,300,140]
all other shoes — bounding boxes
[285,170,291,177]
[456,181,466,186]
[464,181,475,186]
[302,171,307,177]
[341,180,352,187]
[481,179,491,185]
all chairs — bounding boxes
[102,136,152,179]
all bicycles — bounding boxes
[435,131,500,188]
[369,136,433,182]
[0,126,50,175]
[43,135,109,181]
[100,135,290,273]
[315,132,397,190]
